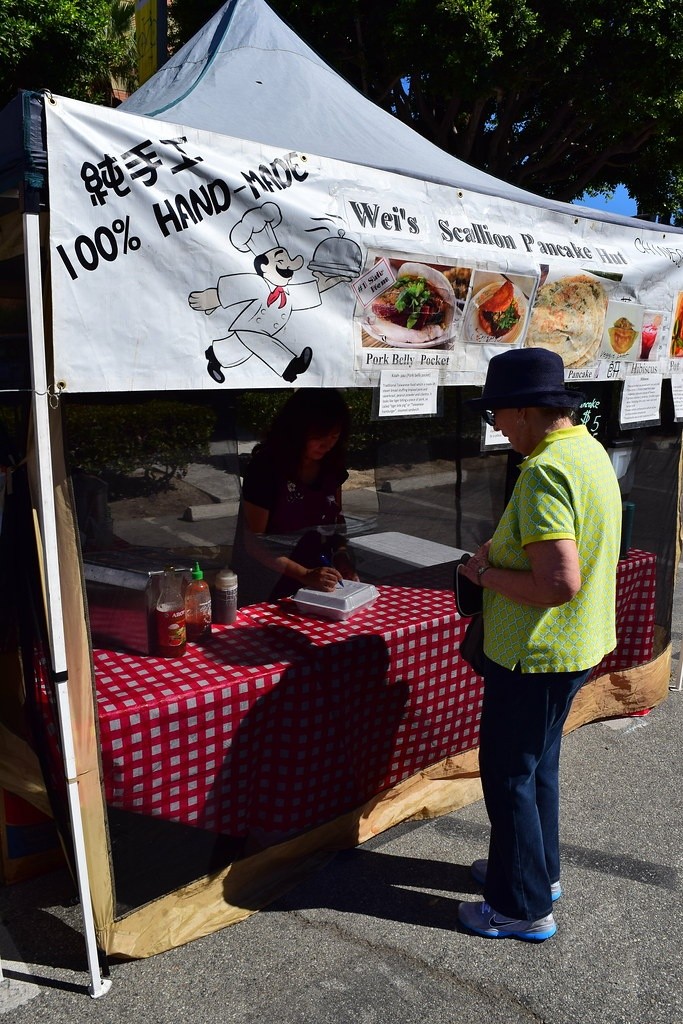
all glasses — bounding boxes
[481,410,495,426]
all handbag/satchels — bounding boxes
[460,615,497,678]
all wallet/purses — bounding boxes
[454,553,483,617]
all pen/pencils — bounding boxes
[319,552,344,587]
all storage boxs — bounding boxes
[288,578,381,621]
[79,545,199,657]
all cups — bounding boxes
[620,501,636,559]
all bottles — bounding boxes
[183,564,214,641]
[158,568,187,656]
[215,562,239,622]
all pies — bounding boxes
[523,275,608,368]
[479,280,521,338]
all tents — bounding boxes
[0,0,683,1002]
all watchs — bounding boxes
[477,565,492,588]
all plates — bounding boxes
[466,282,524,344]
[361,303,463,348]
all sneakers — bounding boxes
[459,900,557,940]
[471,859,561,902]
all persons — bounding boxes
[443,348,623,942]
[228,387,360,609]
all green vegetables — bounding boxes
[393,275,430,329]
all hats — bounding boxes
[464,348,586,408]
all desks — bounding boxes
[348,531,476,587]
[24,539,657,965]
